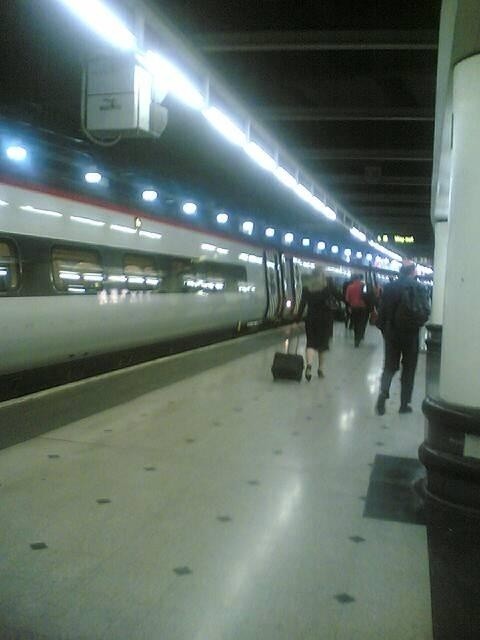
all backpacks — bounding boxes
[392,279,432,337]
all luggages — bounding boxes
[272,327,304,386]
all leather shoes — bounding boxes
[398,404,412,411]
[376,395,385,416]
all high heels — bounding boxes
[304,363,312,380]
[317,367,323,382]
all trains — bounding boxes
[0,175,433,398]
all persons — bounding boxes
[376,261,432,415]
[295,265,353,381]
[340,271,433,349]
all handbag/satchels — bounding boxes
[362,284,377,303]
